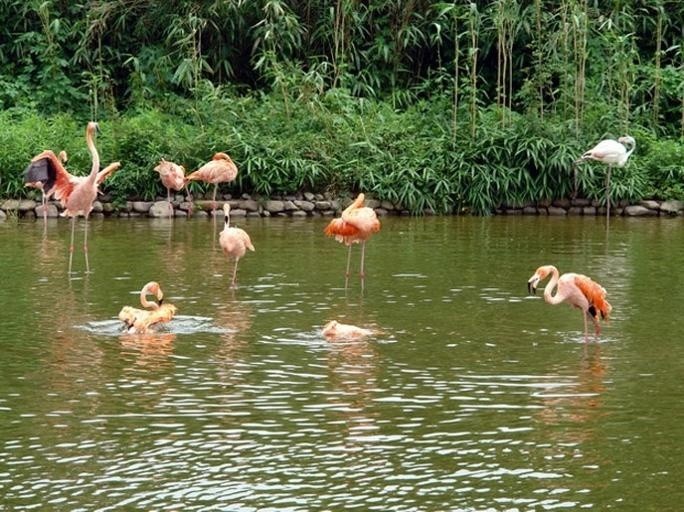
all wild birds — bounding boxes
[23,150,67,219]
[187,153,238,201]
[117,280,177,335]
[322,319,368,341]
[574,133,636,231]
[217,202,254,289]
[527,264,611,341]
[153,157,192,219]
[24,122,121,272]
[322,192,381,298]
